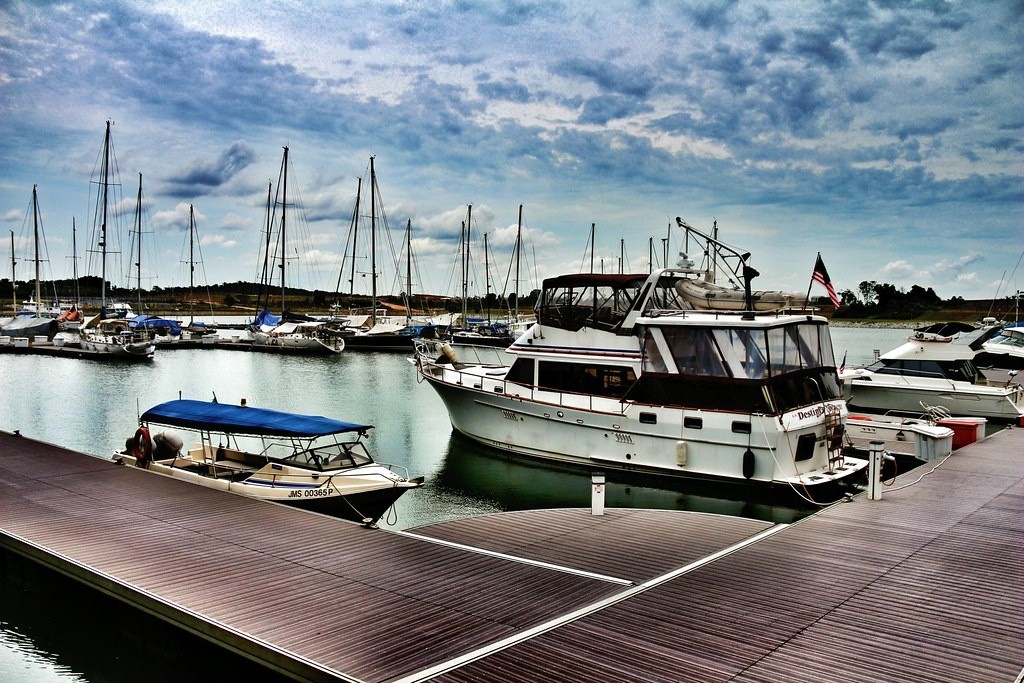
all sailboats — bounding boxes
[323,153,541,352]
[248,140,352,354]
[0,117,184,349]
[179,204,218,334]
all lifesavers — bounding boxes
[272,339,277,345]
[675,278,786,310]
[134,428,151,459]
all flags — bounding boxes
[840,356,847,374]
[811,254,840,311]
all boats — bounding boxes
[113,391,427,532]
[677,277,813,309]
[405,210,872,506]
[829,251,1024,424]
